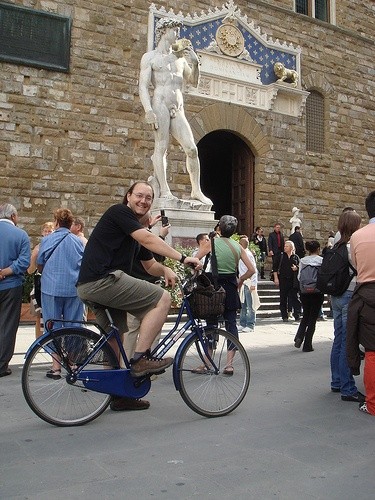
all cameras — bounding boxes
[162,217,169,227]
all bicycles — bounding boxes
[21,262,251,427]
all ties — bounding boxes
[277,233,280,248]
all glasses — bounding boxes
[132,191,153,201]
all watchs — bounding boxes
[180,253,188,262]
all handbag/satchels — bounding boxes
[33,271,40,307]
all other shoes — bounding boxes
[236,325,245,331]
[283,318,288,322]
[331,387,342,393]
[68,362,78,370]
[223,364,234,375]
[261,276,266,279]
[0,368,12,377]
[288,312,292,317]
[190,364,213,375]
[242,327,254,333]
[46,369,61,379]
[316,317,328,321]
[358,401,369,413]
[341,390,366,402]
[294,343,301,348]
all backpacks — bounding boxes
[314,239,358,297]
[181,269,225,314]
[300,264,321,294]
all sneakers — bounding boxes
[129,349,174,377]
[110,395,150,411]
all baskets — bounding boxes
[190,289,226,319]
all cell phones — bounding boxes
[161,210,165,217]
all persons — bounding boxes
[251,226,268,279]
[191,230,259,333]
[76,182,203,411]
[121,209,172,381]
[318,207,357,321]
[0,203,31,379]
[26,206,90,380]
[139,17,214,206]
[295,241,324,352]
[350,191,375,416]
[289,226,306,259]
[330,213,367,402]
[191,215,255,375]
[273,240,305,325]
[288,206,304,238]
[269,224,284,281]
